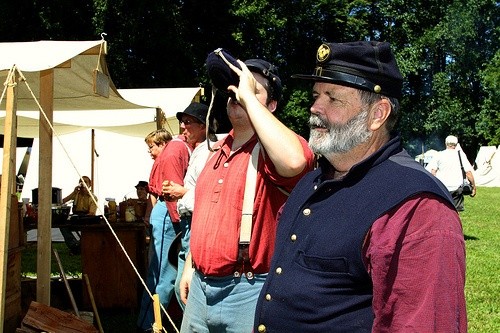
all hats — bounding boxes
[243,58,283,101]
[176,102,216,135]
[135,180,148,189]
[291,40,404,102]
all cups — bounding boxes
[106,198,116,223]
[125,206,135,223]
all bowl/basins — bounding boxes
[36,206,71,224]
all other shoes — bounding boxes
[70,245,81,256]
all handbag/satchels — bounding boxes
[461,183,473,195]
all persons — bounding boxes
[180,57,316,333]
[423,133,476,214]
[254,41,468,333]
[148,132,195,333]
[59,176,98,257]
[145,127,173,160]
[135,181,149,199]
[162,102,222,312]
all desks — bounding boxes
[23,220,145,312]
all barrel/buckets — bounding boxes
[81,230,134,311]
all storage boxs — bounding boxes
[4,194,22,333]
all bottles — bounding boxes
[207,52,242,104]
